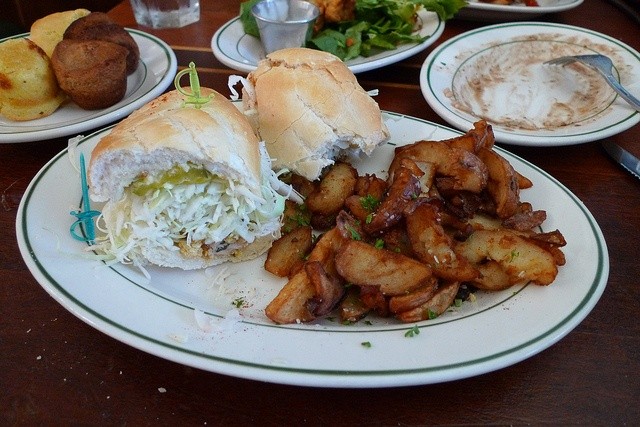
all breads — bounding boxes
[52,39,130,109]
[85,88,281,270]
[0,38,66,121]
[63,13,140,72]
[242,48,391,182]
[29,9,91,58]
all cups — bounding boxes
[251,2,320,57]
[131,0,200,29]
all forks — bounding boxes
[542,54,640,112]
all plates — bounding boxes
[211,8,447,76]
[15,99,610,389]
[0,24,179,143]
[420,21,640,147]
[464,0,584,13]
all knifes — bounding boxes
[602,141,640,179]
